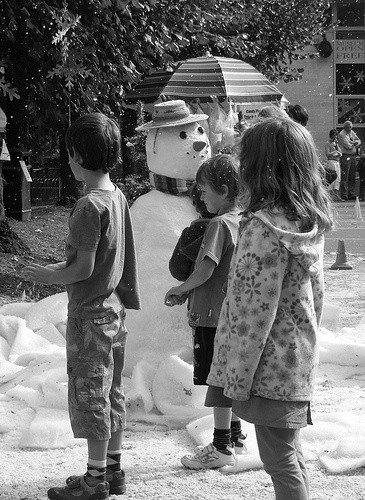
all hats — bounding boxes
[134,99,211,132]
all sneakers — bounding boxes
[65,469,124,494]
[47,474,109,500]
[180,443,235,470]
[194,434,247,455]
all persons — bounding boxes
[165,105,361,469]
[205,118,333,500]
[19,113,141,500]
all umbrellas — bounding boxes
[124,50,284,105]
